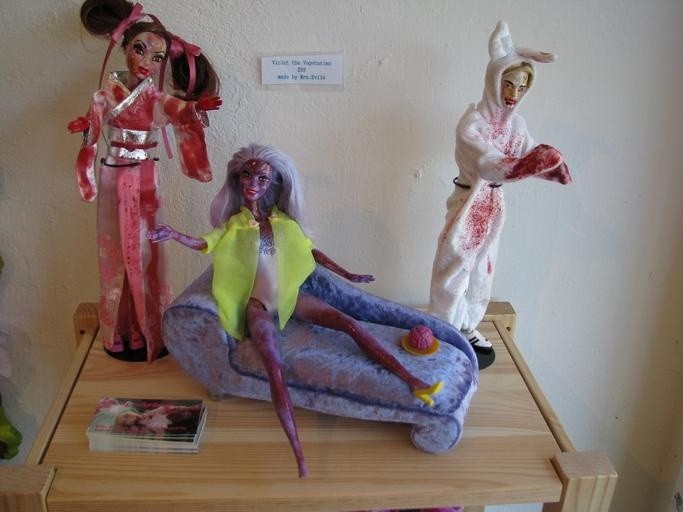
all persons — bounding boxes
[148,144,443,478]
[65,1,574,482]
[430,17,571,348]
[70,2,224,353]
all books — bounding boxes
[87,397,207,453]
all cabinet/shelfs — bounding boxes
[0,300,618,512]
